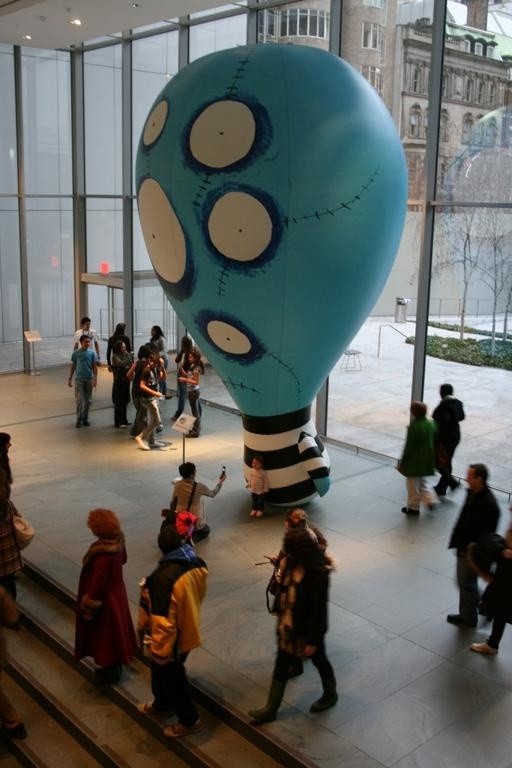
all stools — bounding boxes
[340,349,363,370]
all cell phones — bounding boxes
[219,465,226,480]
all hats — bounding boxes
[88,508,123,538]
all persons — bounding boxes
[398,400,439,514]
[247,529,338,723]
[471,493,512,654]
[265,508,328,680]
[432,383,465,497]
[447,464,500,628]
[247,456,270,518]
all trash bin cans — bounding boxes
[395,297,408,323]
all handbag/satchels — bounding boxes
[175,511,198,535]
[12,514,34,549]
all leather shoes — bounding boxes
[402,507,420,518]
[0,722,27,738]
[448,613,477,626]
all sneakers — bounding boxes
[161,717,205,737]
[83,419,89,425]
[248,706,275,719]
[137,700,155,713]
[249,510,256,515]
[75,422,81,427]
[312,694,337,713]
[256,510,263,516]
[186,432,198,437]
[119,420,164,449]
[472,641,497,653]
